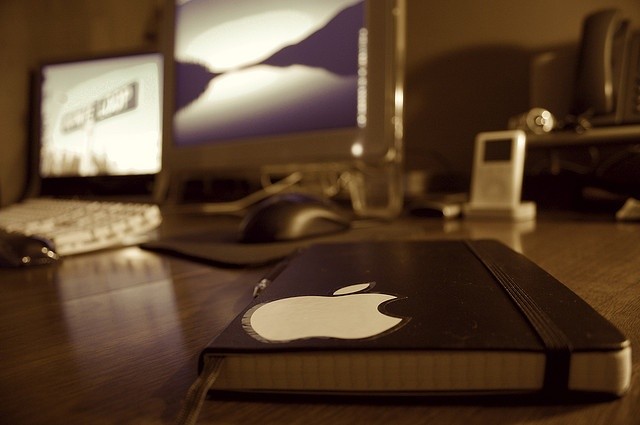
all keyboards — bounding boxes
[1,197,164,258]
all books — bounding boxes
[173,236,632,425]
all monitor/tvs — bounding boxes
[36,50,164,199]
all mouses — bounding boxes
[235,192,351,244]
